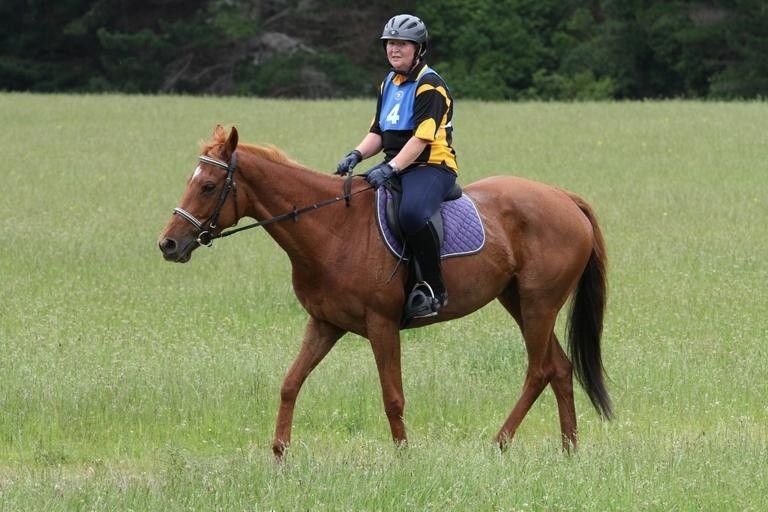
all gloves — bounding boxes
[336,150,363,175]
[365,161,397,191]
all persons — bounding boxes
[336,13,458,318]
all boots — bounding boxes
[407,220,448,309]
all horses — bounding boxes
[157,125,623,458]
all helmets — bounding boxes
[380,14,429,60]
